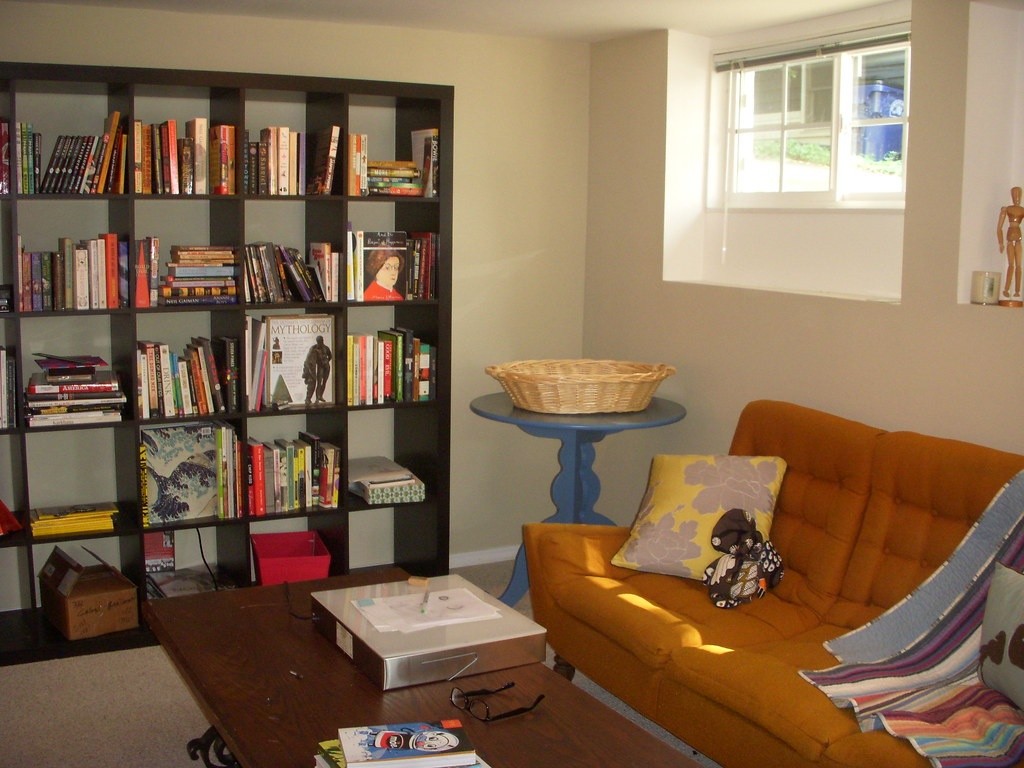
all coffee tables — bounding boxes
[141,566,702,768]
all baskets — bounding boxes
[484,358,675,414]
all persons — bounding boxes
[997,187,1024,296]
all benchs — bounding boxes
[519,399,1024,768]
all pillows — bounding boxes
[977,560,1024,712]
[610,453,789,585]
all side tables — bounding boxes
[468,391,690,609]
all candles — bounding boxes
[970,270,1002,303]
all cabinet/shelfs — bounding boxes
[0,60,457,671]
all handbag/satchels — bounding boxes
[703,508,785,608]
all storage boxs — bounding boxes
[307,572,547,689]
[251,530,330,587]
[35,545,138,642]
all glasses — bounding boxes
[449,682,546,722]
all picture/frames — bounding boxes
[265,314,339,412]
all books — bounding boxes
[0,112,127,194]
[245,242,339,302]
[139,421,242,526]
[23,355,127,427]
[0,345,17,430]
[143,530,226,598]
[137,336,238,419]
[0,499,118,536]
[350,129,439,198]
[347,221,437,301]
[247,431,341,515]
[347,328,436,405]
[0,233,129,312]
[245,313,336,410]
[245,126,340,194]
[135,117,235,195]
[136,236,237,307]
[348,456,415,486]
[314,719,490,768]
